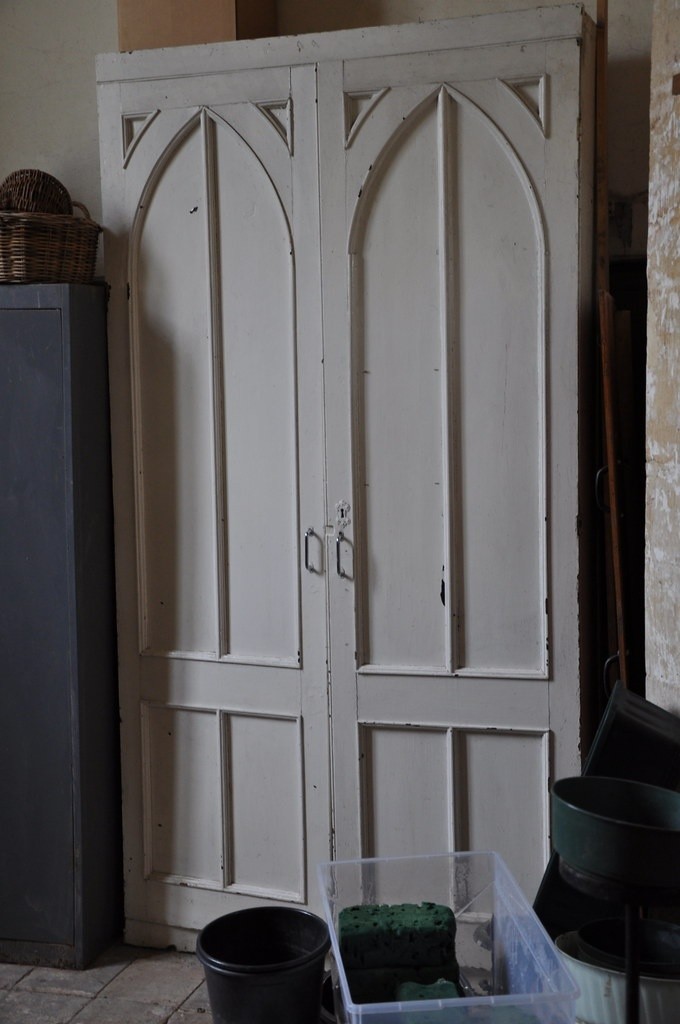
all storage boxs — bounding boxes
[117,0,275,52]
[315,850,580,1024]
[530,679,680,943]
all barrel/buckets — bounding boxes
[196,907,330,1024]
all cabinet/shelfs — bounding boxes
[96,3,599,956]
[0,282,123,971]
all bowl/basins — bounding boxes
[550,777,680,886]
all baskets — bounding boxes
[1,201,101,284]
[2,168,74,216]
